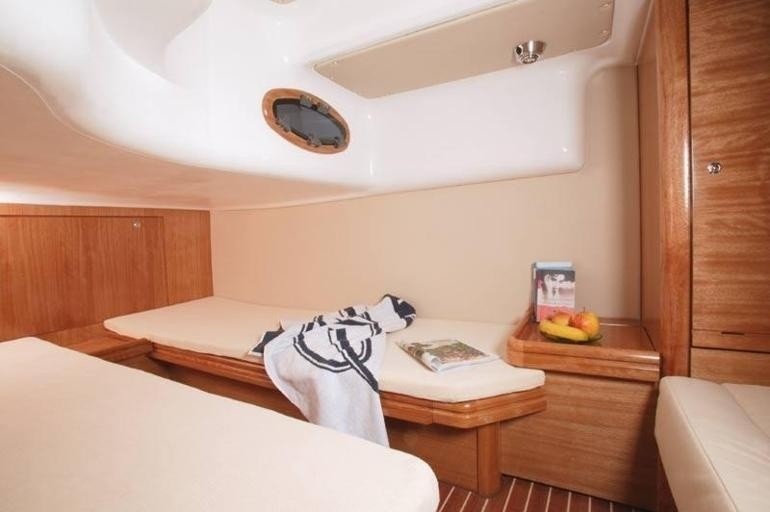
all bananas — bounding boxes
[539,319,589,341]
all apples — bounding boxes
[571,312,600,338]
[550,312,570,328]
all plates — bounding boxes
[538,330,604,345]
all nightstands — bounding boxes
[499,317,662,507]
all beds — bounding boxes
[0,336,441,511]
[104,295,545,498]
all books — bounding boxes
[531,258,577,326]
[398,336,500,374]
